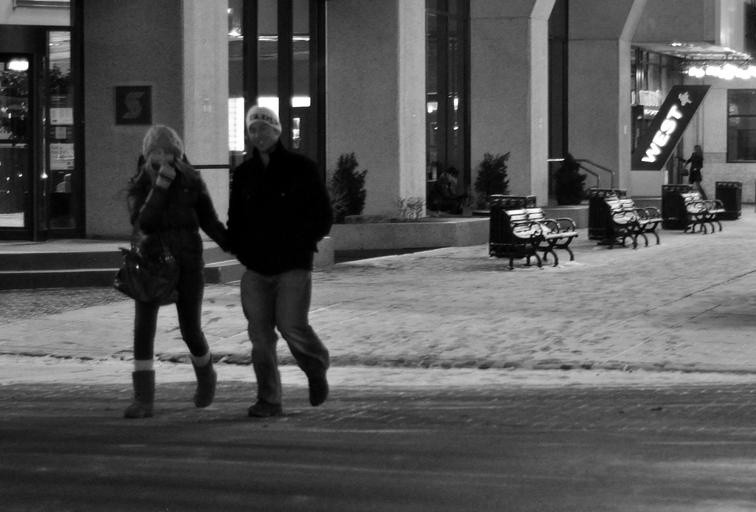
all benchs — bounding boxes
[604,197,665,249]
[500,207,577,270]
[678,191,726,235]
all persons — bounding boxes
[224,104,332,419]
[123,122,236,419]
[683,145,709,202]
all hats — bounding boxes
[245,105,282,134]
[143,123,183,161]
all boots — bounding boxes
[123,370,156,419]
[306,365,329,408]
[247,395,285,418]
[186,352,221,409]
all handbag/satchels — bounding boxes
[114,206,181,306]
